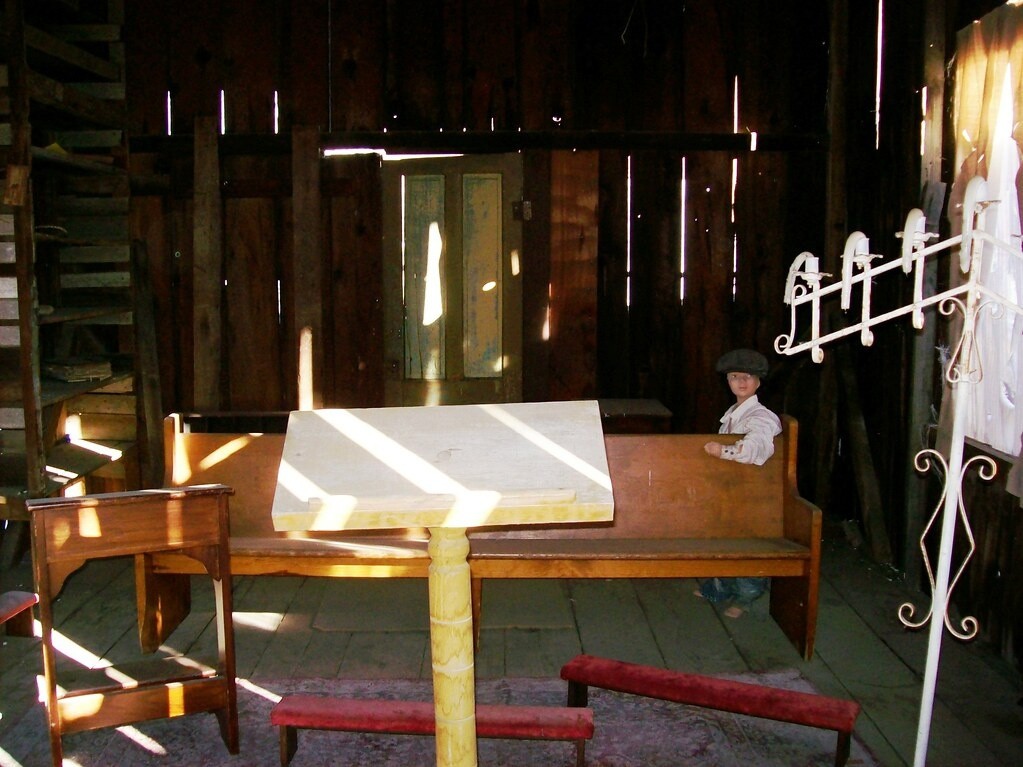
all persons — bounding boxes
[694,351,781,619]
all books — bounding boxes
[46,357,111,380]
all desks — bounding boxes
[271,399,616,767]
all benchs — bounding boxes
[270,695,595,767]
[0,591,39,643]
[560,655,862,767]
[136,412,823,662]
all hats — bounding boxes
[718,349,768,378]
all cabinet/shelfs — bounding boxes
[1,0,169,579]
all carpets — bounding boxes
[0,666,885,767]
